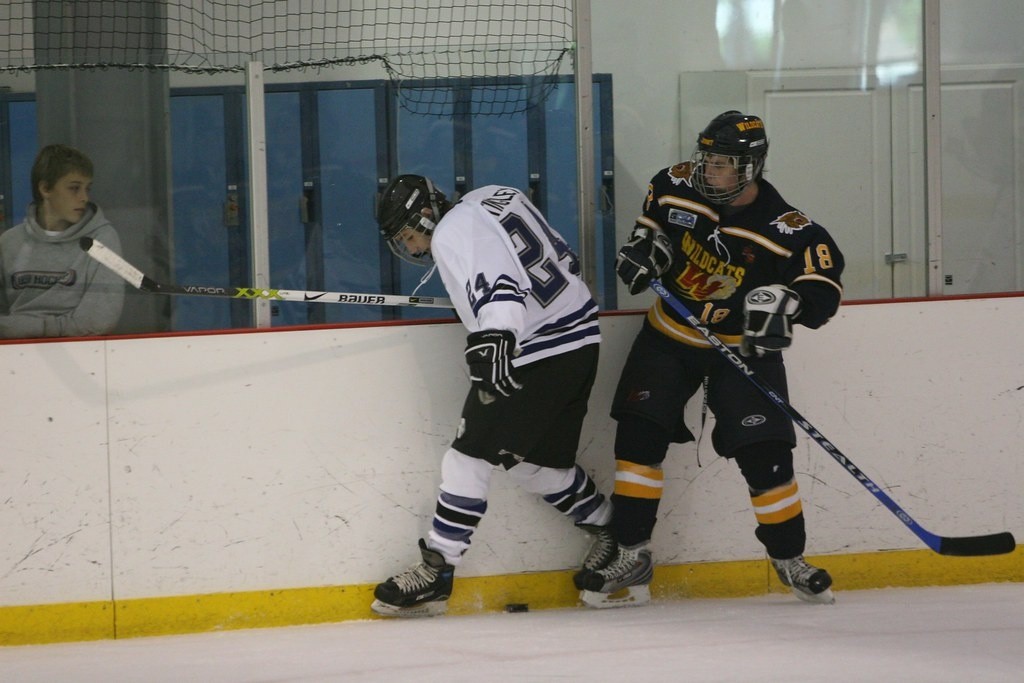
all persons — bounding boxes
[370,176,619,617]
[578,110,845,610]
[0,144,125,338]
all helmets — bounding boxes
[378,174,448,268]
[692,110,767,203]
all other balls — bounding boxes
[504,604,529,613]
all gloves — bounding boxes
[742,285,804,350]
[614,226,671,293]
[464,330,522,401]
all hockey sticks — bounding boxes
[647,276,1020,559]
[78,232,460,313]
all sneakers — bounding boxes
[581,543,654,607]
[573,493,616,590]
[371,538,454,614]
[769,555,837,608]
